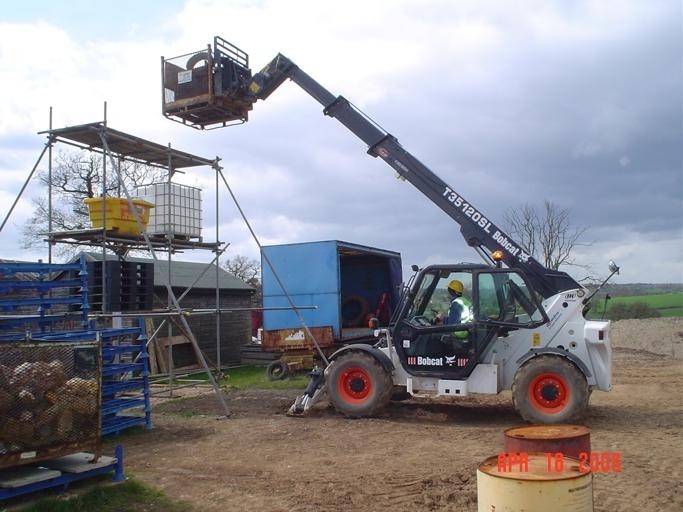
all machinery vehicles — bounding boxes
[161,36,619,425]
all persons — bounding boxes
[433,280,474,343]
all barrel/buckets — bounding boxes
[475,453,595,512]
[505,424,591,459]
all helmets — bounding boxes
[446,280,464,292]
